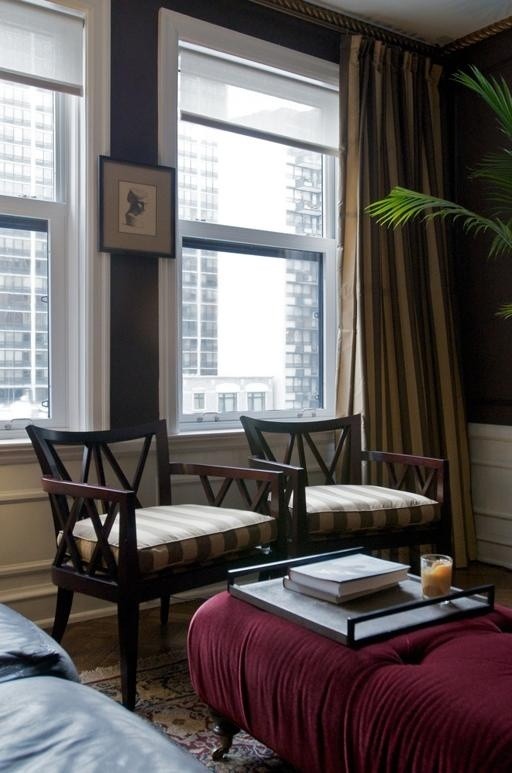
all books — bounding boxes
[288,553,411,598]
[282,575,399,604]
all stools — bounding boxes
[187,583,511,773]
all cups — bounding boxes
[420,553,454,606]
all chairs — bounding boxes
[26,420,284,711]
[240,414,457,591]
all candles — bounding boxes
[424,559,452,593]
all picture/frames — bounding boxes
[96,155,174,258]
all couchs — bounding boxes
[0,599,207,773]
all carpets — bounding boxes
[80,647,303,773]
